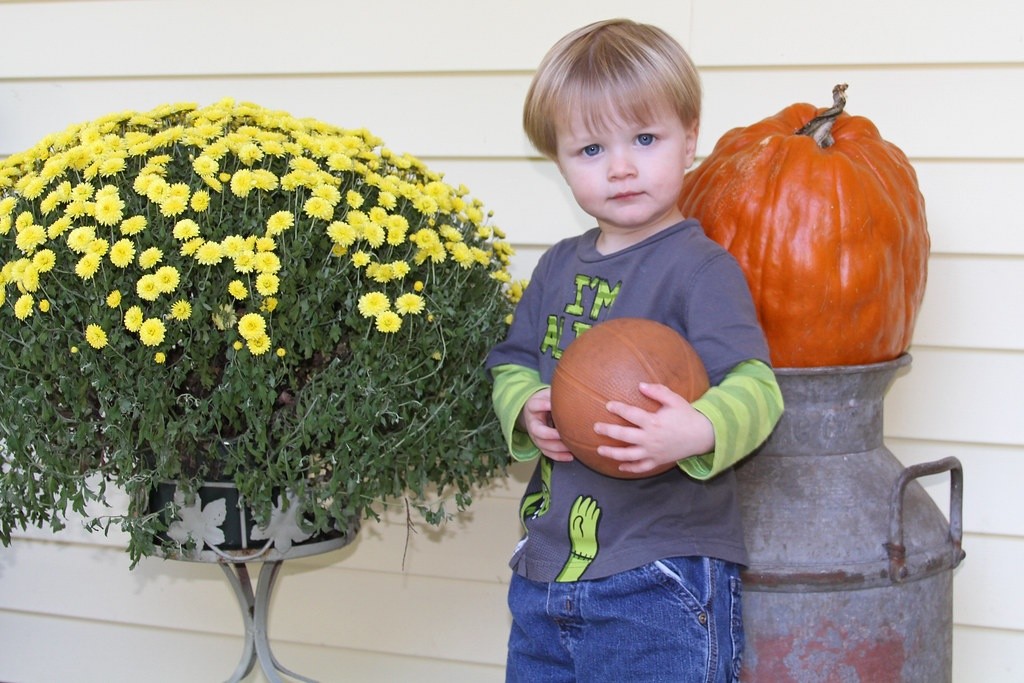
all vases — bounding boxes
[128,428,360,566]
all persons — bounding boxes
[484,18,785,683]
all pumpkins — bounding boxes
[676,81,932,368]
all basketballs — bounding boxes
[549,318,710,480]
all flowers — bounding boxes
[0,94,530,572]
